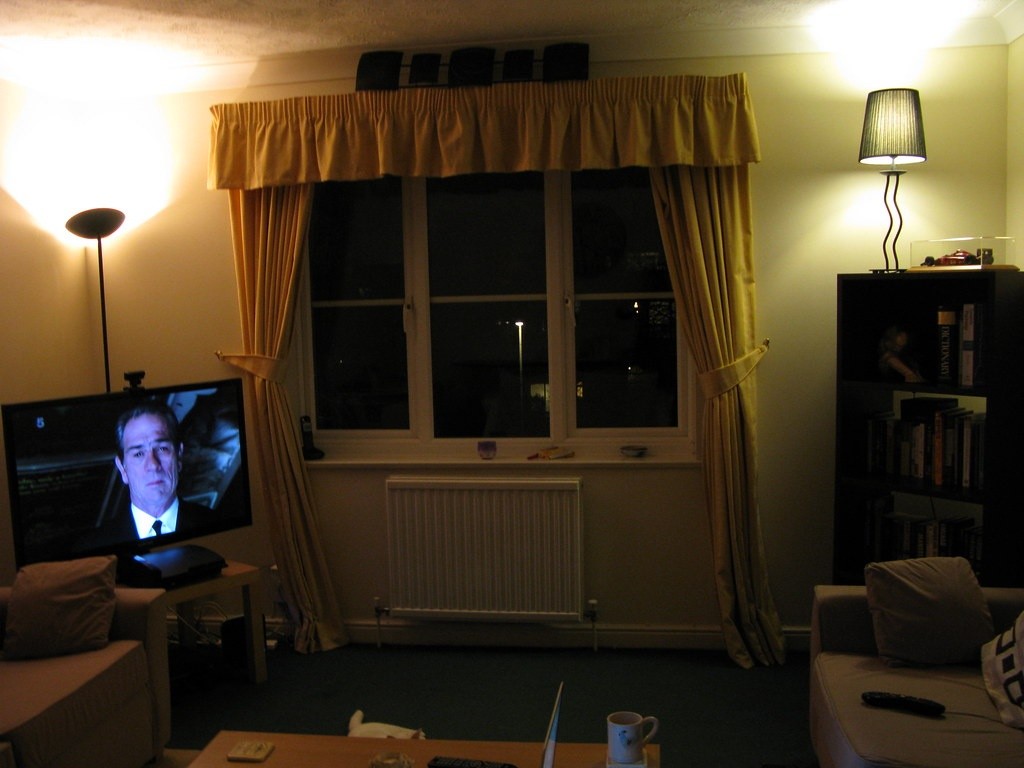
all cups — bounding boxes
[607,711,659,764]
[477,441,497,459]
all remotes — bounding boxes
[428,756,519,768]
[861,692,945,716]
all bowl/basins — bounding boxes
[620,445,648,456]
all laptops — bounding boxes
[540,681,565,768]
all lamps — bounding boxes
[66,206,126,393]
[860,88,926,271]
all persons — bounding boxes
[70,404,220,555]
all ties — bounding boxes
[152,521,163,536]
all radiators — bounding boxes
[385,476,585,625]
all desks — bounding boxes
[134,561,270,697]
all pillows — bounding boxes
[5,554,119,656]
[866,557,993,667]
[980,605,1024,730]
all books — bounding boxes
[861,303,986,576]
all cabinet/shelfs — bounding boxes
[833,268,1024,584]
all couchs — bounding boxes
[807,583,1024,768]
[0,583,171,767]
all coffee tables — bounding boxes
[180,729,659,768]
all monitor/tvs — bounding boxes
[0,378,252,589]
